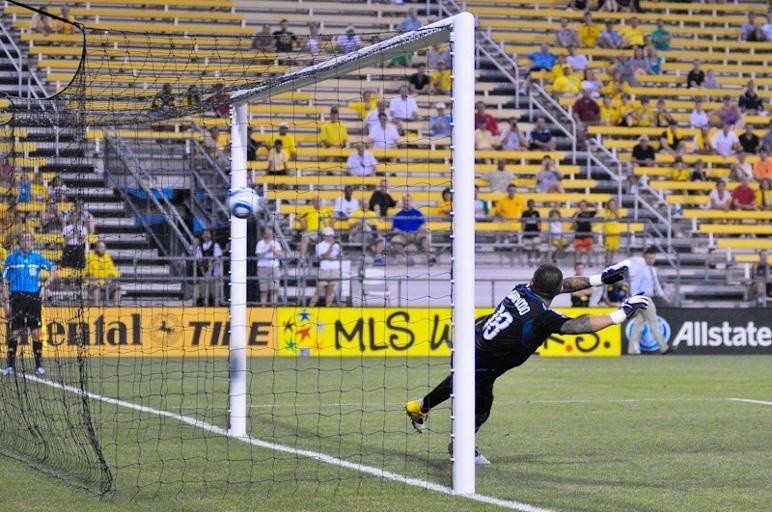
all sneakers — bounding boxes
[3,367,15,376]
[35,368,45,379]
[405,400,429,434]
[448,442,491,465]
[428,258,436,266]
[374,260,387,265]
[407,258,413,266]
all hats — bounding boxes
[323,227,335,236]
[436,102,445,109]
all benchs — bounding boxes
[1,1,771,309]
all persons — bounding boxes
[605,244,671,355]
[0,157,122,307]
[404,263,650,465]
[150,1,772,307]
[1,231,55,375]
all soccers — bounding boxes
[228,187,262,218]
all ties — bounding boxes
[650,267,658,300]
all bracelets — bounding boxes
[609,309,626,324]
[43,283,49,290]
[588,273,602,286]
[3,297,10,303]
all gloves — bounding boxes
[589,266,628,288]
[610,290,648,326]
